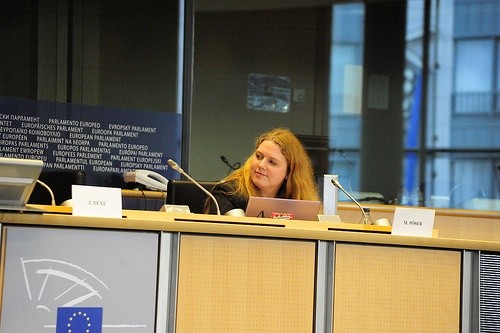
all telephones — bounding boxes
[123,169,169,191]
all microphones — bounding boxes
[221,155,241,175]
[167,159,221,215]
[331,178,368,224]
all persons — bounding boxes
[204,127,323,216]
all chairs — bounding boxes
[166,180,220,214]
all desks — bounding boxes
[0,211,500,333]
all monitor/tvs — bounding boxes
[0,157,44,205]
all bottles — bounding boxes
[359,207,374,225]
[393,184,423,206]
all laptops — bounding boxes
[245,197,321,220]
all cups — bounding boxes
[430,195,450,208]
